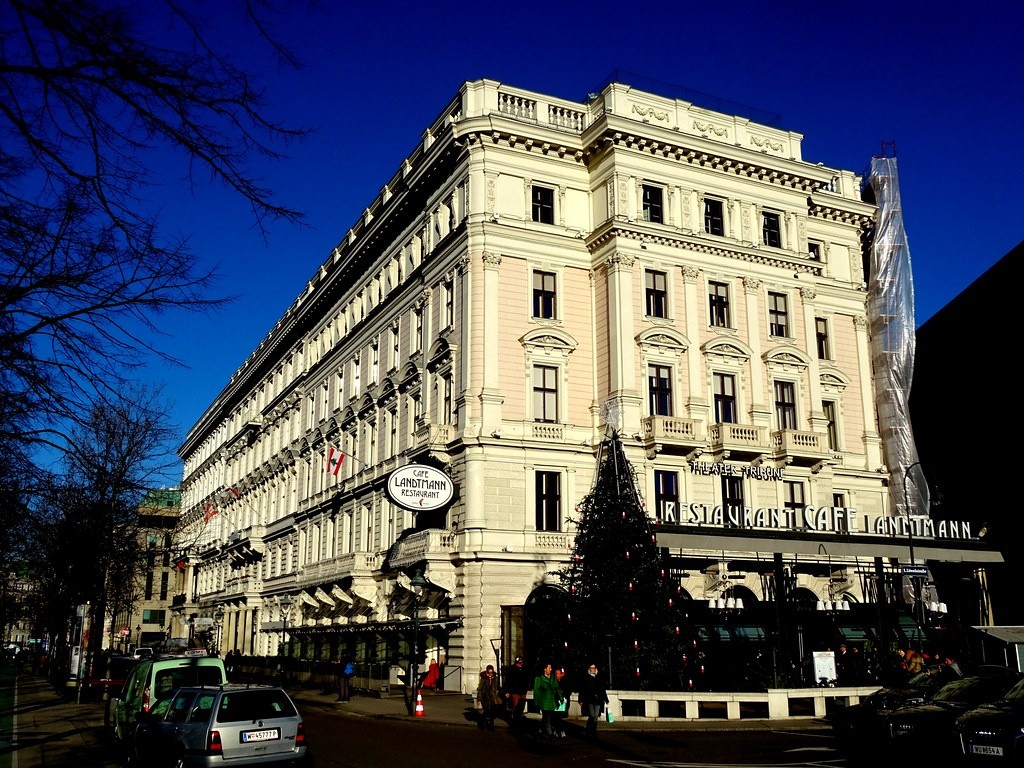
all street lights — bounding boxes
[409,570,426,713]
[135,624,141,647]
[904,462,945,673]
[278,594,293,691]
[215,608,224,656]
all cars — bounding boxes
[953,677,1024,768]
[831,664,1024,768]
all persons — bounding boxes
[421,659,439,688]
[478,666,500,732]
[828,643,962,678]
[336,649,353,702]
[504,656,530,726]
[534,662,608,739]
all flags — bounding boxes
[327,446,346,477]
[229,485,242,498]
[203,502,218,523]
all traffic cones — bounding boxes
[414,689,426,717]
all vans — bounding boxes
[70,646,81,681]
[134,647,154,660]
[112,655,229,745]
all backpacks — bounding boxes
[345,663,353,675]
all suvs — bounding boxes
[129,681,307,768]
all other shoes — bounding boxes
[335,698,348,704]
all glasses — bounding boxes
[488,672,494,674]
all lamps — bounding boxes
[912,558,947,623]
[815,557,850,622]
[708,551,744,623]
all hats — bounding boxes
[840,643,847,649]
[515,658,522,663]
